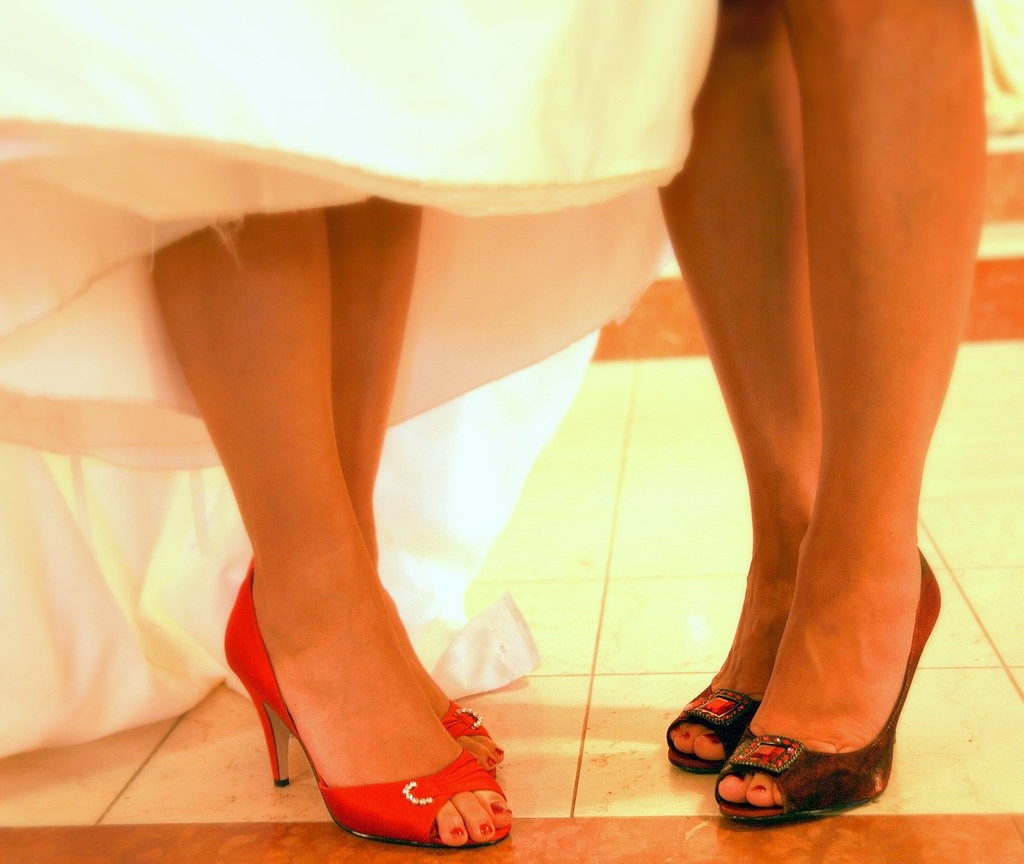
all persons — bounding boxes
[658,0,987,821]
[1,0,718,850]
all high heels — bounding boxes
[442,701,503,780]
[225,552,513,850]
[715,547,940,825]
[666,685,766,777]
